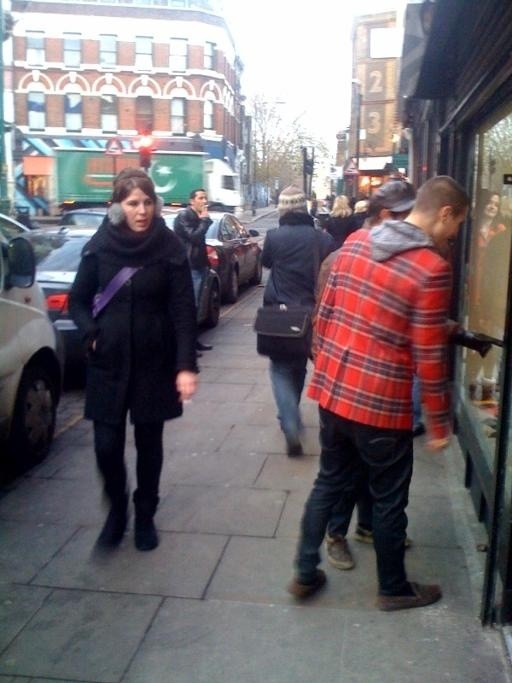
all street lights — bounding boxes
[349,77,364,203]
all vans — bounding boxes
[0,231,67,493]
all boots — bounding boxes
[99,493,159,551]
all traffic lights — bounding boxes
[305,158,314,179]
[140,127,153,170]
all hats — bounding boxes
[278,186,307,216]
[377,181,416,212]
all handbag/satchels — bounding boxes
[256,308,311,355]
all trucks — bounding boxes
[51,147,243,216]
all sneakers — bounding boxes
[326,531,411,570]
[378,584,442,610]
[288,571,325,596]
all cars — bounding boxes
[8,224,221,381]
[0,208,264,307]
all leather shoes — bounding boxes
[196,343,212,356]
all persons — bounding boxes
[173,188,213,359]
[67,166,200,562]
[256,179,504,609]
